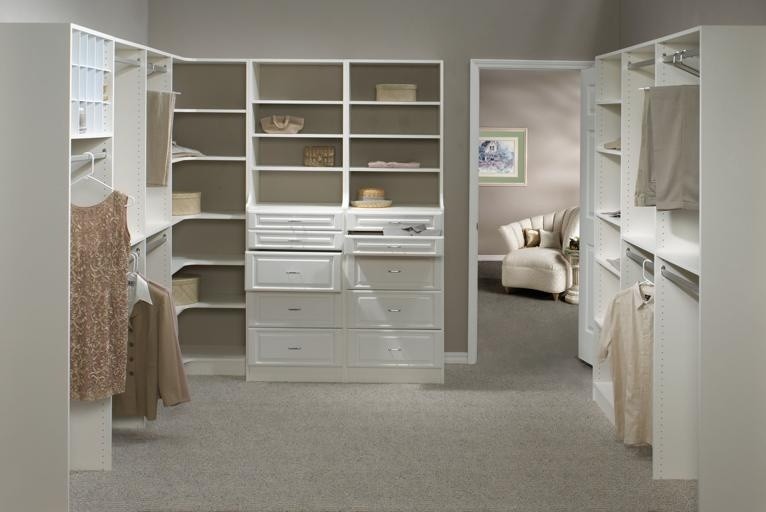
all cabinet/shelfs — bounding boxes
[593,25,766,512]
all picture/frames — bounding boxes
[479,126,528,187]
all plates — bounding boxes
[350,199,393,208]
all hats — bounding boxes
[350,187,393,208]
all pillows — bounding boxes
[524,229,562,248]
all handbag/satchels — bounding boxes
[304,146,336,167]
[260,115,305,134]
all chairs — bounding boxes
[497,207,580,302]
